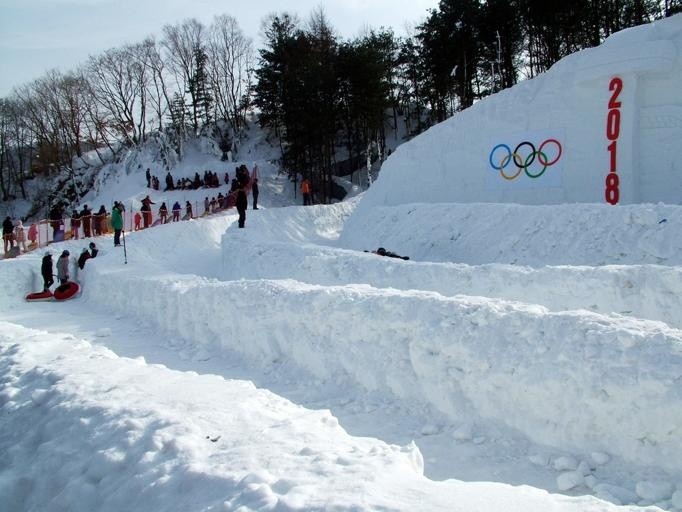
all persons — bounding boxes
[1,164,259,292]
[302,178,313,205]
